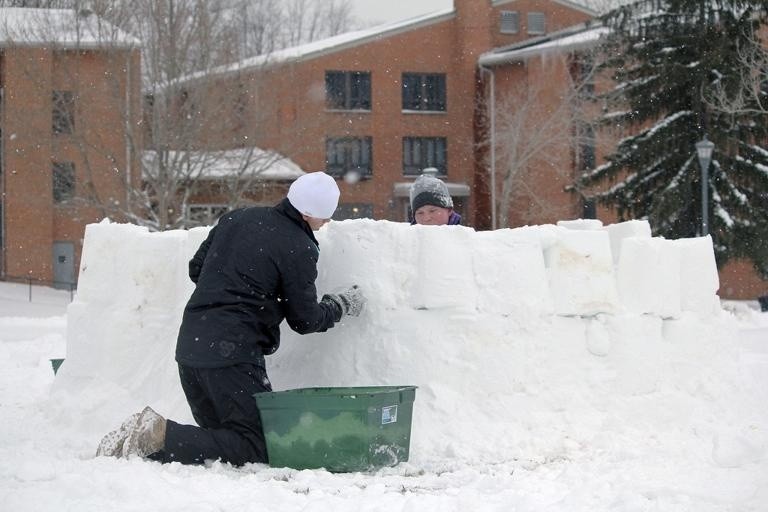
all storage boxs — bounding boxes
[253,381,416,474]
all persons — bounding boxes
[409,173,462,226]
[94,171,369,468]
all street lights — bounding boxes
[694,132,716,237]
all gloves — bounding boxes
[322,286,368,322]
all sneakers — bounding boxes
[123,407,166,460]
[96,413,141,459]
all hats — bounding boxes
[287,171,340,219]
[409,175,453,215]
